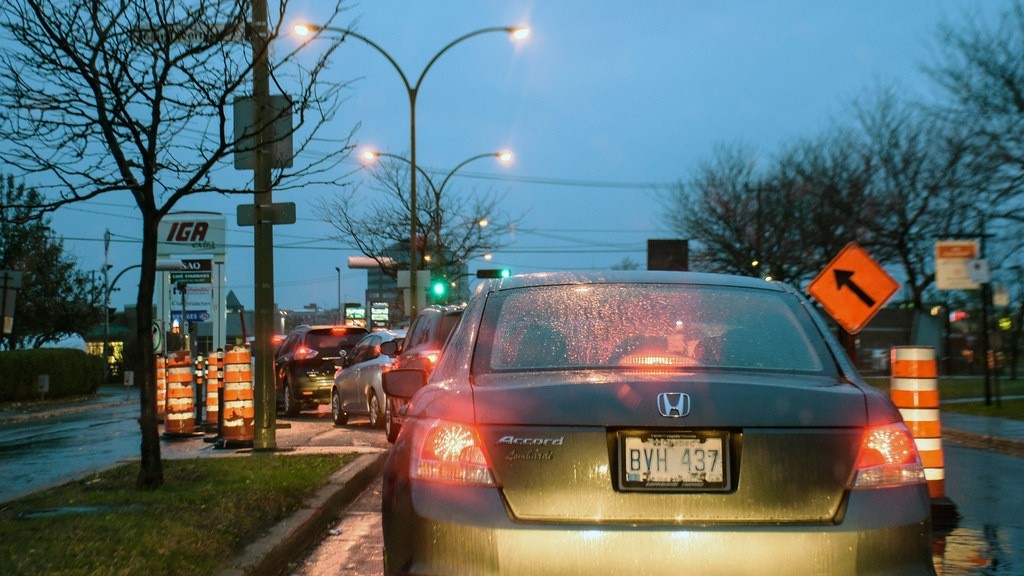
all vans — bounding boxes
[246,336,287,393]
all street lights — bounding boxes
[296,24,531,327]
[335,267,341,323]
[361,151,512,267]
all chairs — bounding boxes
[504,324,569,367]
[610,333,670,367]
[693,328,754,367]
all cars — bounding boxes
[381,274,936,576]
[331,326,409,428]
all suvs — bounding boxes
[274,324,370,419]
[385,306,466,441]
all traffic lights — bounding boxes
[477,270,511,279]
[434,282,444,305]
